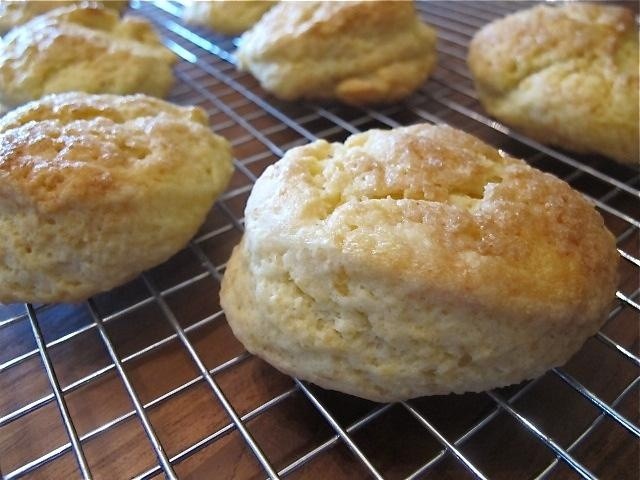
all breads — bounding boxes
[186,0,437,105]
[465,0,640,166]
[218,123,618,395]
[0,1,230,303]
